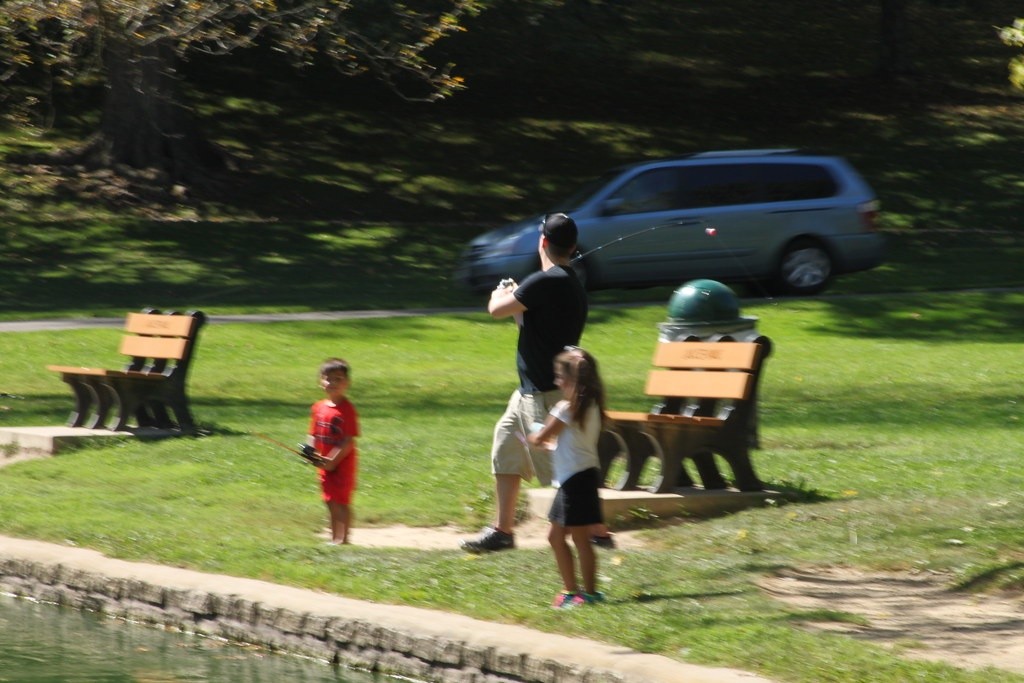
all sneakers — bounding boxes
[461,525,514,552]
[589,534,614,548]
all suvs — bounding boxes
[451,149,903,301]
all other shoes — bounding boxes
[552,590,604,607]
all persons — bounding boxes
[459,212,616,554]
[300,357,360,547]
[527,346,605,608]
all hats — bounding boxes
[538,213,578,241]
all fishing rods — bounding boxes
[255,433,328,468]
[500,215,713,289]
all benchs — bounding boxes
[47,306,209,431]
[600,341,769,491]
